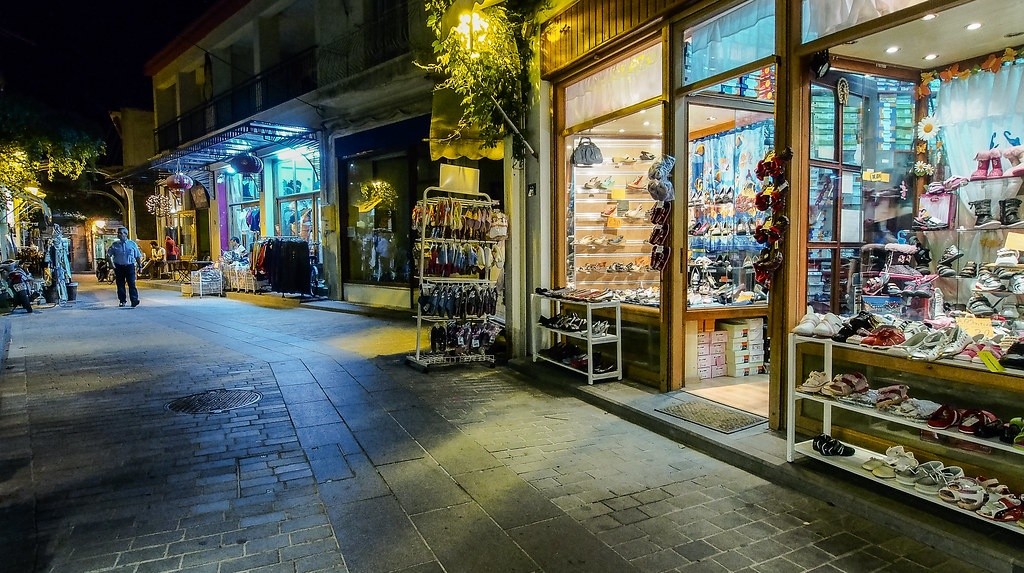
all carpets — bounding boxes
[655,400,770,434]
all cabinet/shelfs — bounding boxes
[222,264,261,296]
[407,186,498,368]
[191,270,223,299]
[529,293,622,385]
[787,332,1024,536]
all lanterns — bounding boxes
[231,152,264,181]
[167,172,193,205]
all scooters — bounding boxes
[0,258,33,313]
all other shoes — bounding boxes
[578,150,676,307]
[686,145,795,306]
[131,300,139,307]
[119,302,125,306]
[863,146,1024,317]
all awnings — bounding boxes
[0,183,51,225]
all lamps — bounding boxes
[811,49,831,81]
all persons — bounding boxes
[229,237,248,257]
[165,236,177,272]
[250,206,261,233]
[239,207,253,232]
[106,227,144,308]
[140,241,167,280]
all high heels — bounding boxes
[535,286,616,374]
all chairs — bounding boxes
[171,257,196,283]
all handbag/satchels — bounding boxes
[570,137,602,164]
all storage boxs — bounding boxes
[697,317,764,378]
[811,77,940,205]
[919,193,957,230]
[684,41,776,100]
[807,271,825,296]
[860,295,929,322]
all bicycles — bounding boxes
[97,258,115,285]
[49,266,68,307]
[308,243,320,297]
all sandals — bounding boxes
[791,311,1024,521]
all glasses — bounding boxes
[117,232,124,234]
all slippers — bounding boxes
[411,200,508,355]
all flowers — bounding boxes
[913,113,944,176]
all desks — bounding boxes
[165,260,191,283]
[189,261,215,271]
